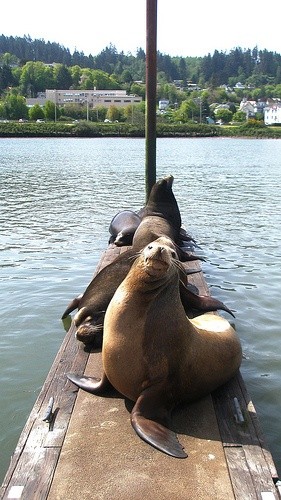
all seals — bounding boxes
[61,174,242,459]
